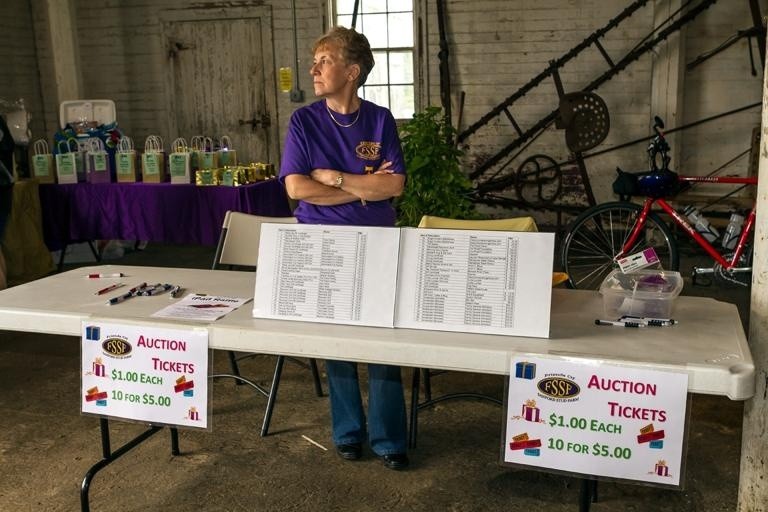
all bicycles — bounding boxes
[560,116,759,289]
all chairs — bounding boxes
[209,210,326,438]
[405,214,540,452]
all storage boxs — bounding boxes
[598,267,686,321]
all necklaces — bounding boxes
[327,106,361,128]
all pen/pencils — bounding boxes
[94,283,123,296]
[110,282,181,305]
[85,272,123,279]
[595,315,680,329]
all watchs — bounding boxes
[335,172,344,190]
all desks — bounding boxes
[36,175,286,271]
[1,263,756,512]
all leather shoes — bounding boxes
[337,443,364,461]
[383,454,408,470]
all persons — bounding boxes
[276,26,411,472]
